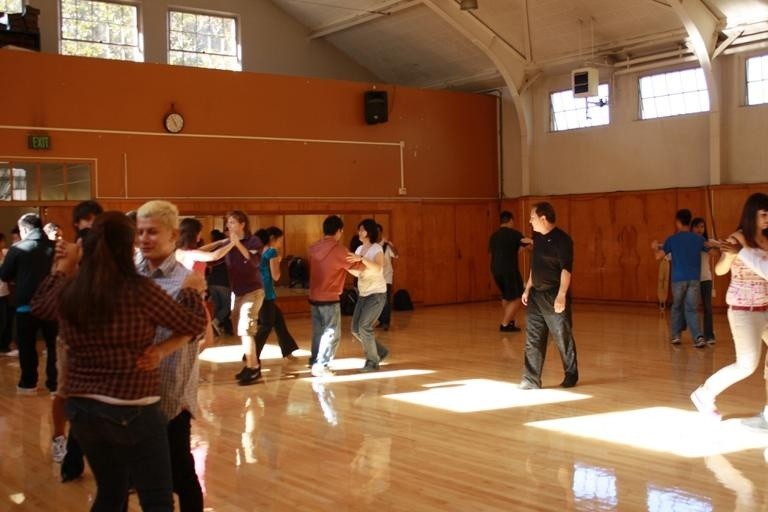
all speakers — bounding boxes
[363,91,388,125]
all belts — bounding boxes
[732,305,767,312]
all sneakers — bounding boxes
[48,388,57,395]
[500,320,521,332]
[235,364,262,384]
[358,345,388,372]
[211,319,222,337]
[671,336,716,347]
[690,384,722,420]
[281,357,336,377]
[17,384,38,390]
[560,373,578,388]
[52,435,84,481]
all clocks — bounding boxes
[163,103,184,133]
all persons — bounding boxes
[372,225,400,331]
[691,194,767,424]
[305,216,364,379]
[488,211,533,330]
[0,199,300,512]
[652,208,714,348]
[516,202,578,391]
[720,231,768,282]
[346,220,387,373]
[657,217,722,344]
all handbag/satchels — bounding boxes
[340,289,357,316]
[289,257,309,289]
[392,289,413,312]
